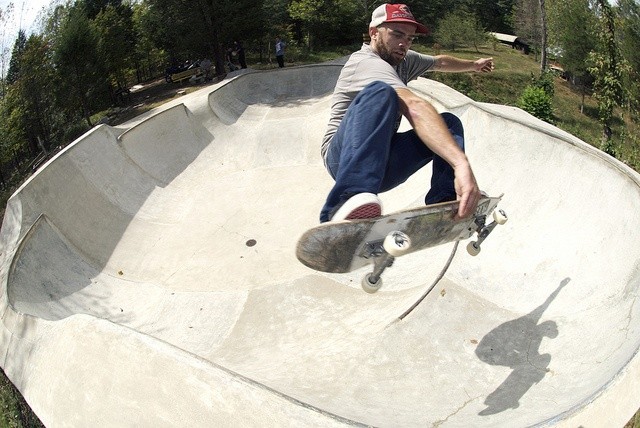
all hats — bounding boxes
[369,3,428,34]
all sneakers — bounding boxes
[329,192,381,222]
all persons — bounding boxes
[320,3,495,223]
[233,40,247,69]
[226,48,234,64]
[275,37,286,68]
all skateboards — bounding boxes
[294,193,507,293]
